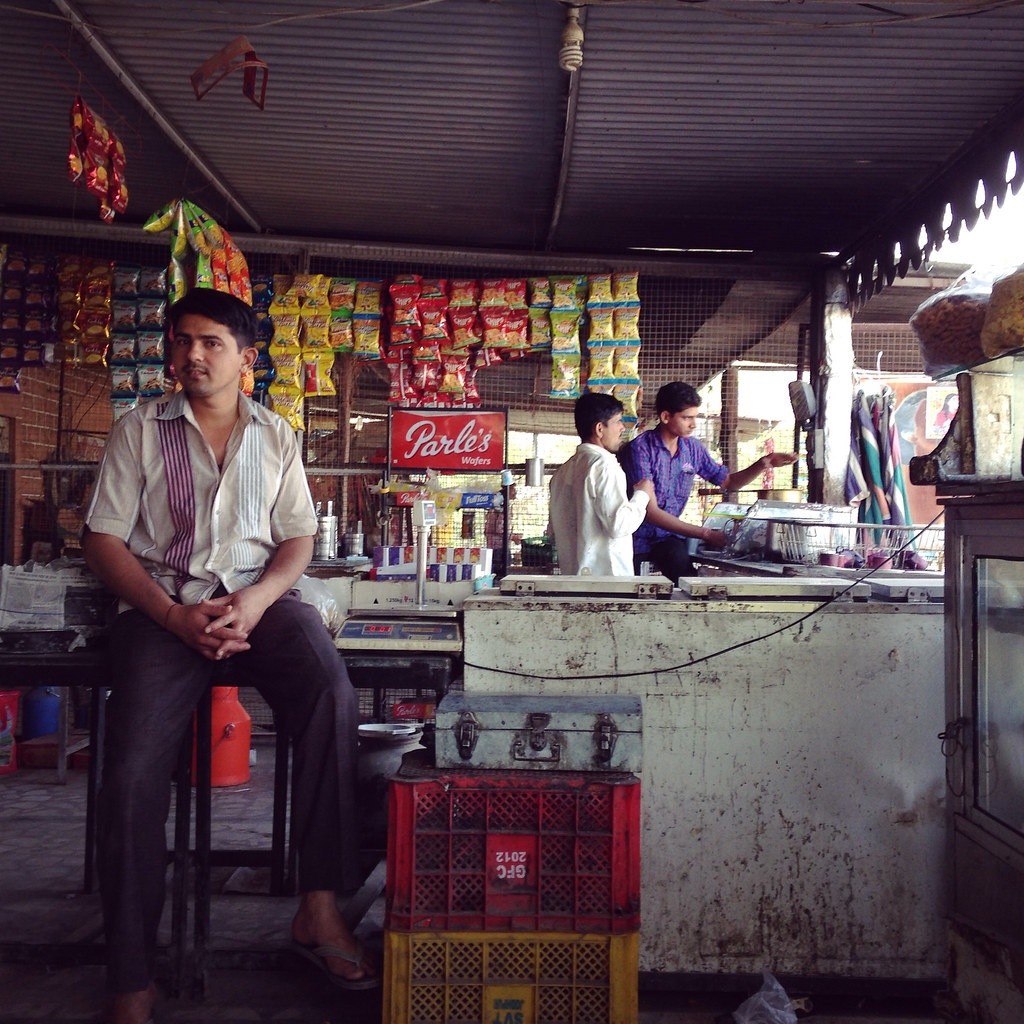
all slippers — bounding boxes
[287,938,383,991]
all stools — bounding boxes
[86,656,288,1000]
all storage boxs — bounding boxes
[384,748,643,934]
[383,932,639,1024]
[373,545,493,582]
[436,690,645,772]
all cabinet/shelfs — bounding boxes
[933,481,1024,1024]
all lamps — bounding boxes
[789,380,817,423]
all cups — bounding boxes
[525,458,544,487]
[873,556,892,569]
[344,533,364,557]
[905,553,928,570]
[501,469,515,486]
[820,553,853,568]
[312,516,338,561]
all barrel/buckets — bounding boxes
[0,690,21,775]
[26,687,62,740]
[192,686,252,787]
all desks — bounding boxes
[0,647,453,893]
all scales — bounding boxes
[332,499,464,653]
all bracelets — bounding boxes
[164,604,180,632]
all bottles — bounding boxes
[355,729,426,848]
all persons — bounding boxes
[616,379,799,588]
[548,391,655,578]
[483,484,522,588]
[77,288,378,1024]
